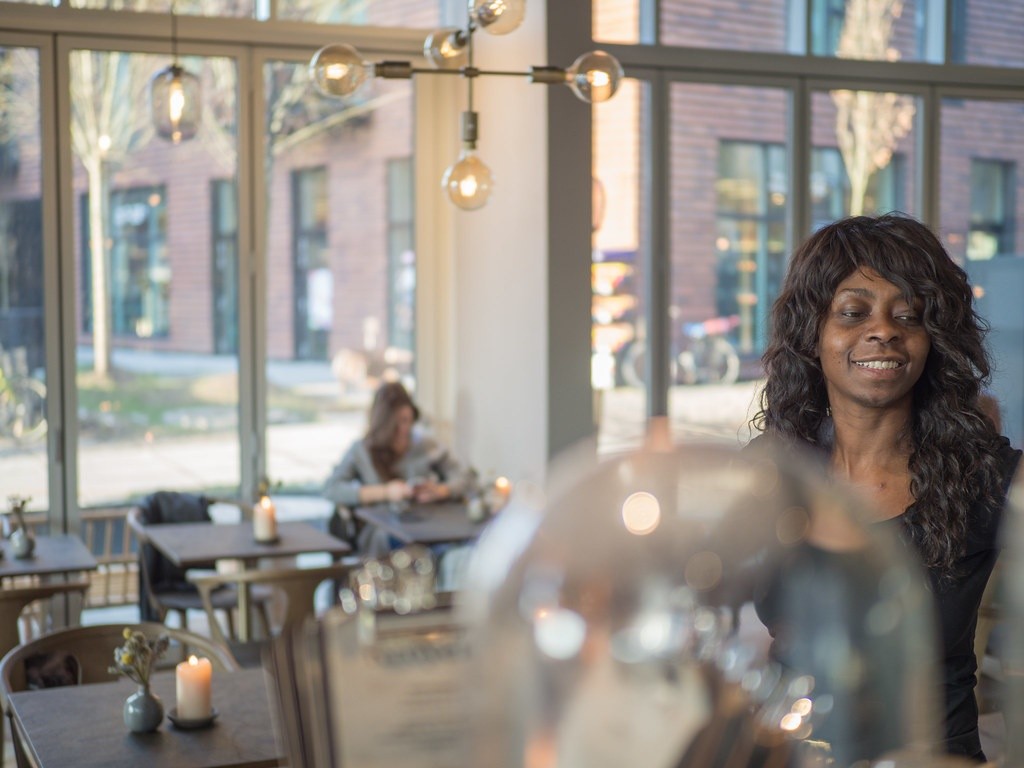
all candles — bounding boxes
[252,496,278,543]
[175,654,214,719]
[490,478,514,518]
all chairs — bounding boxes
[185,561,364,668]
[125,490,273,664]
[0,619,246,768]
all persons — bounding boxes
[319,383,469,574]
[738,210,1018,768]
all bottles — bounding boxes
[10,524,34,559]
[465,493,489,522]
[123,681,164,733]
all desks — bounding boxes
[142,520,352,665]
[352,500,492,560]
[0,533,97,643]
[6,665,281,768]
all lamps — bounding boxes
[138,0,624,213]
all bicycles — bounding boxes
[624,307,737,387]
[0,346,46,444]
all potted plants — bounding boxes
[8,493,35,558]
[109,625,170,738]
[466,469,488,525]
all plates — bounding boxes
[167,705,220,728]
[253,535,281,548]
